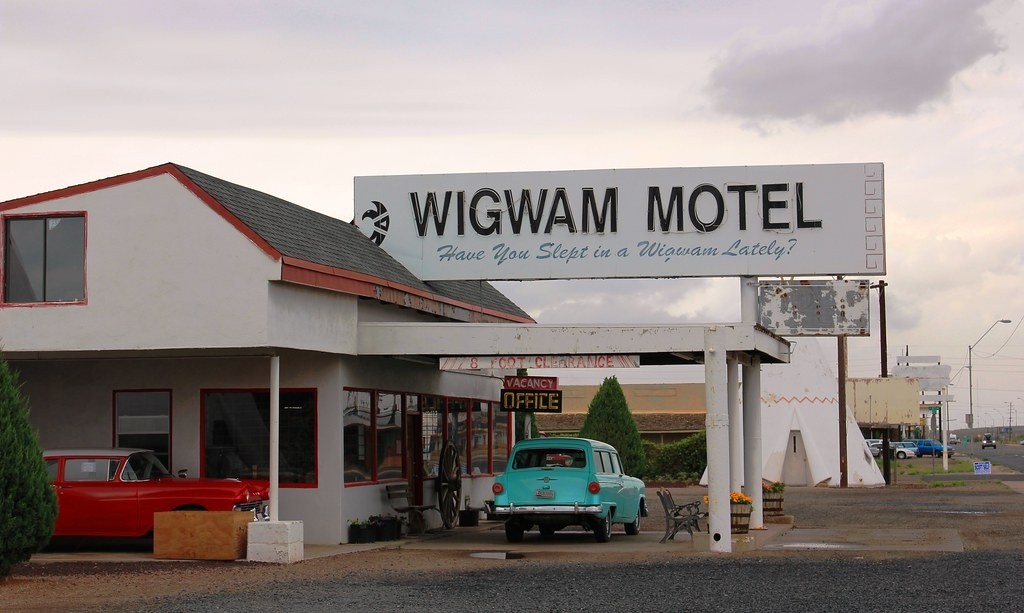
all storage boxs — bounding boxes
[459,510,479,527]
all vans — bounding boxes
[982,433,997,449]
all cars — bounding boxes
[865,439,956,459]
[42,448,270,552]
[492,437,649,543]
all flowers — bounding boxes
[703,492,753,504]
[347,513,411,527]
[761,480,786,492]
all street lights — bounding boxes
[946,366,972,445]
[968,320,1012,458]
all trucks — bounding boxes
[949,433,961,444]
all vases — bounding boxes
[763,492,784,516]
[732,504,753,533]
[350,521,402,543]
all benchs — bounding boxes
[656,489,705,543]
[387,484,445,537]
